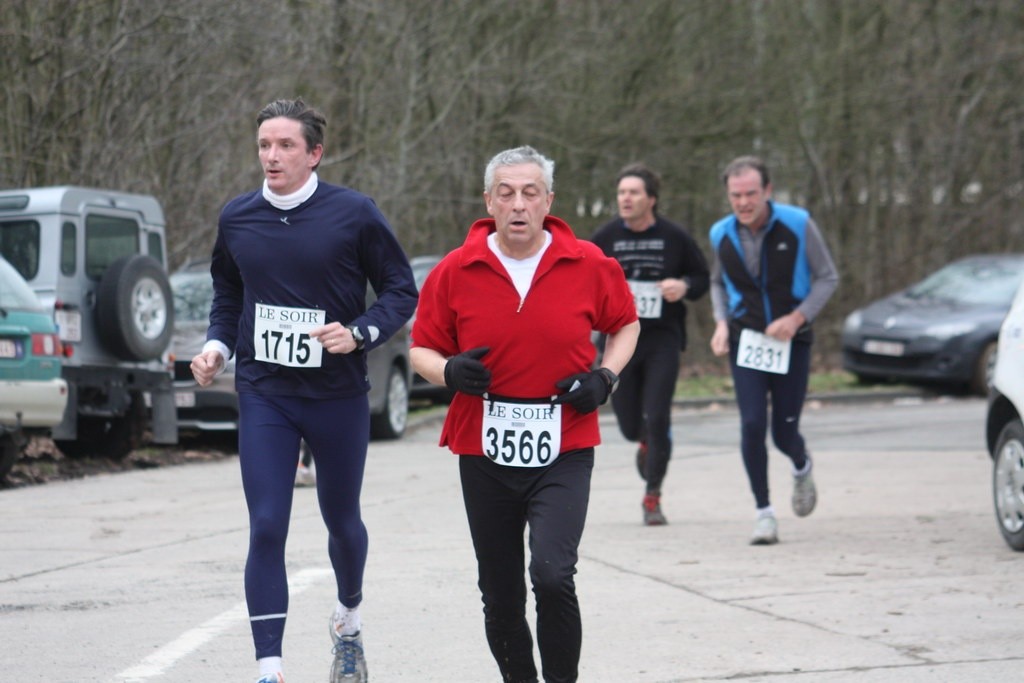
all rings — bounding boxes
[332,339,336,346]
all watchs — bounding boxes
[601,368,620,395]
[347,324,365,348]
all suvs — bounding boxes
[0,185,179,462]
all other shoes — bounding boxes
[642,501,665,524]
[636,441,648,479]
[792,456,816,517]
[749,518,777,545]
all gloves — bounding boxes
[552,368,619,413]
[444,347,491,395]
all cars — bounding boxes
[356,256,455,440]
[0,253,70,486]
[160,265,242,429]
[982,287,1023,552]
[835,252,1024,400]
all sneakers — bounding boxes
[329,612,367,683]
[257,672,283,683]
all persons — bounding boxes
[409,144,640,683]
[588,167,710,526]
[189,99,420,683]
[709,155,840,545]
